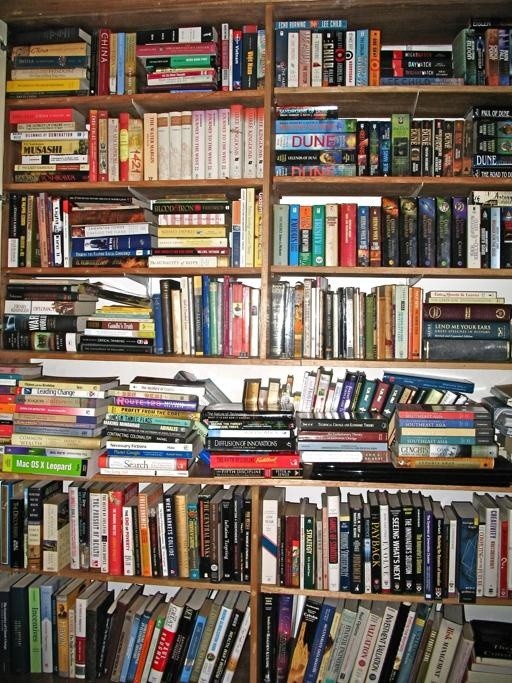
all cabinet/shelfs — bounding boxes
[0,0,512,372]
[1,471,511,682]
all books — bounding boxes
[4,188,262,358]
[1,360,511,477]
[0,19,266,183]
[0,480,511,682]
[275,19,512,177]
[270,192,512,360]
